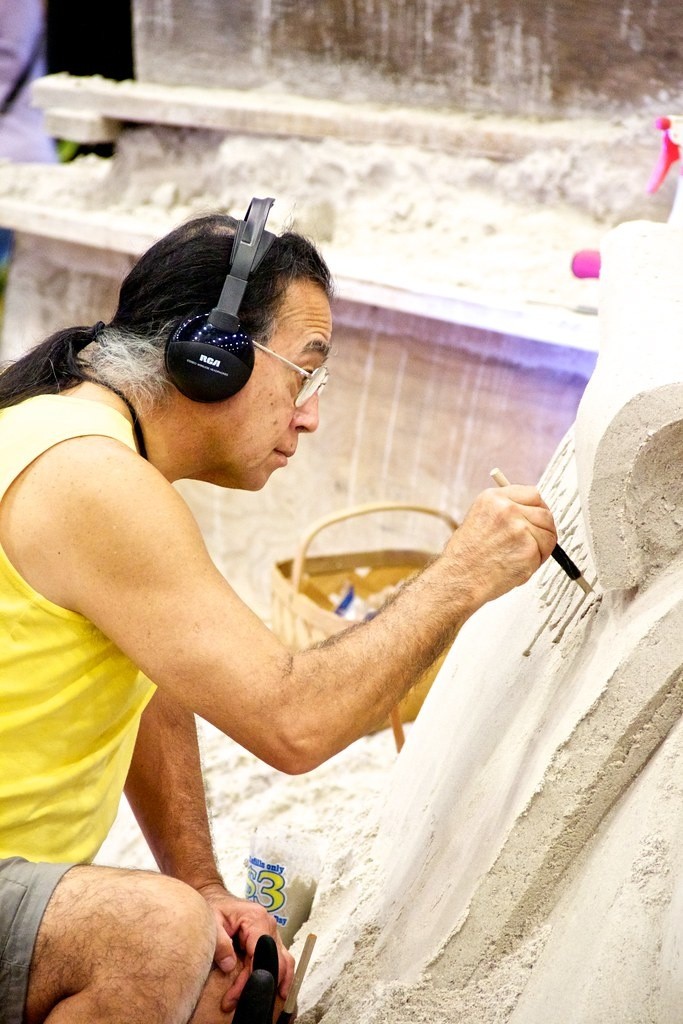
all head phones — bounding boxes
[165,196,279,404]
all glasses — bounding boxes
[255,338,332,408]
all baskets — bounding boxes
[271,502,464,738]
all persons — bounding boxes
[0,0,60,292]
[0,218,556,1024]
